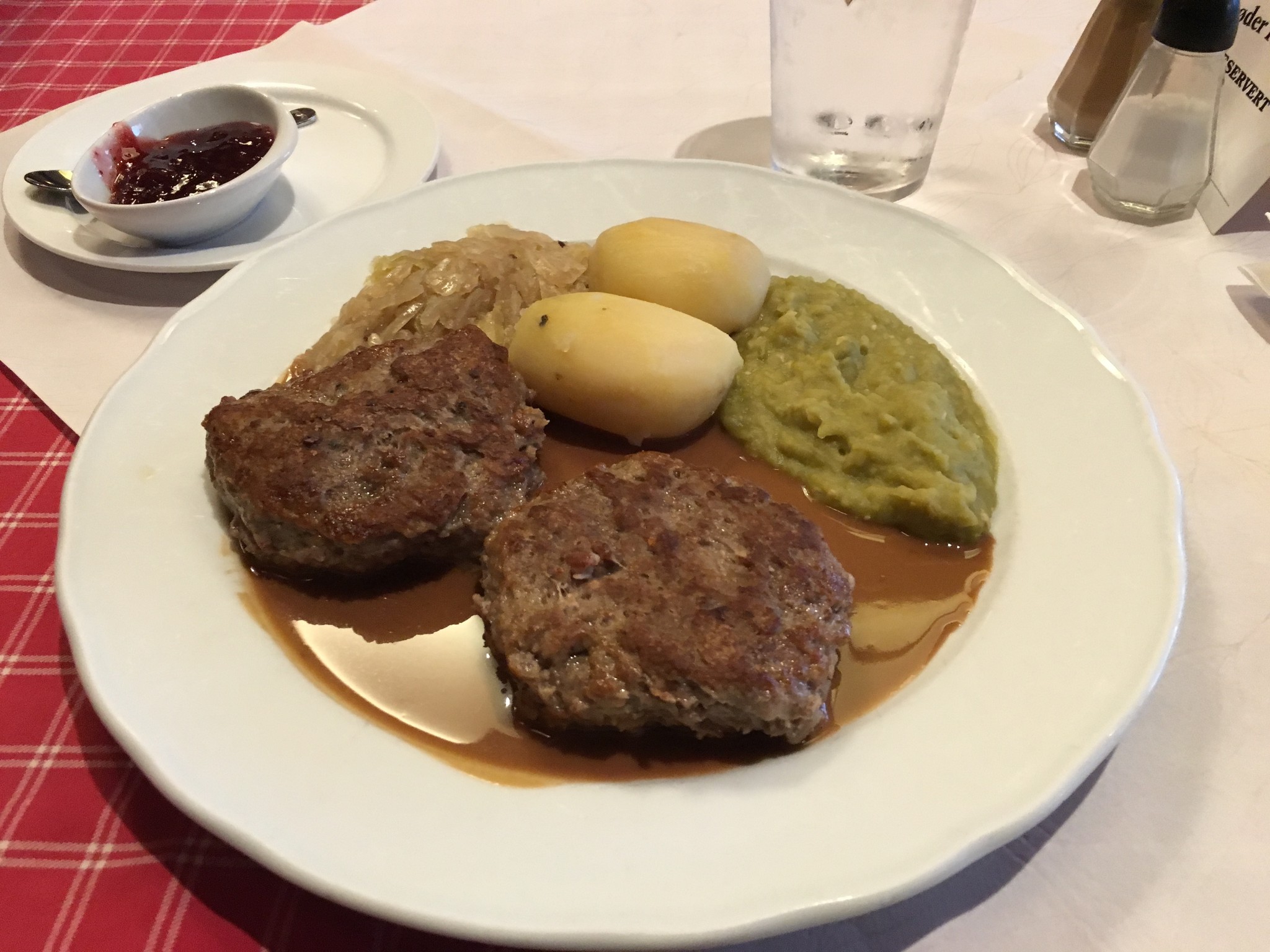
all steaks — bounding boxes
[472,453,855,754]
[198,324,542,584]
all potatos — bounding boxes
[507,218,771,442]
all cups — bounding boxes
[768,1,980,202]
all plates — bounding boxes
[46,155,1188,949]
[2,61,444,276]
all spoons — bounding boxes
[23,104,318,192]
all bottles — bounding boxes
[1045,0,1244,224]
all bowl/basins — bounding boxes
[68,80,300,245]
